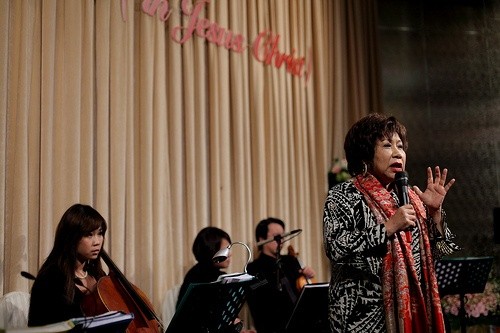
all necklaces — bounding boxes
[74,268,87,278]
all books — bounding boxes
[165,272,255,333]
[284,282,329,333]
[4,311,134,333]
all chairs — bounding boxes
[0,292,31,333]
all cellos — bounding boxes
[81,248,164,333]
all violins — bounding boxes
[287,245,316,290]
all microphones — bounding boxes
[256,229,302,247]
[73,277,97,329]
[394,171,412,243]
[20,271,87,329]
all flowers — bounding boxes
[441,277,500,318]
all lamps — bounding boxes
[212,241,255,283]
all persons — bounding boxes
[323,112,463,333]
[175,226,257,333]
[27,204,107,328]
[244,217,317,333]
